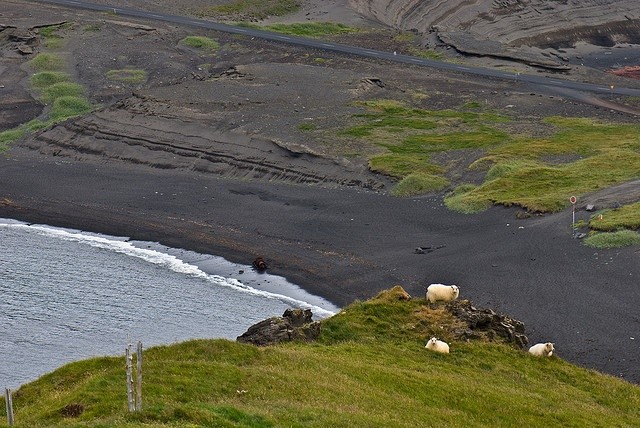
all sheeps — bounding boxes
[529,341,556,358]
[424,337,450,355]
[425,281,460,308]
